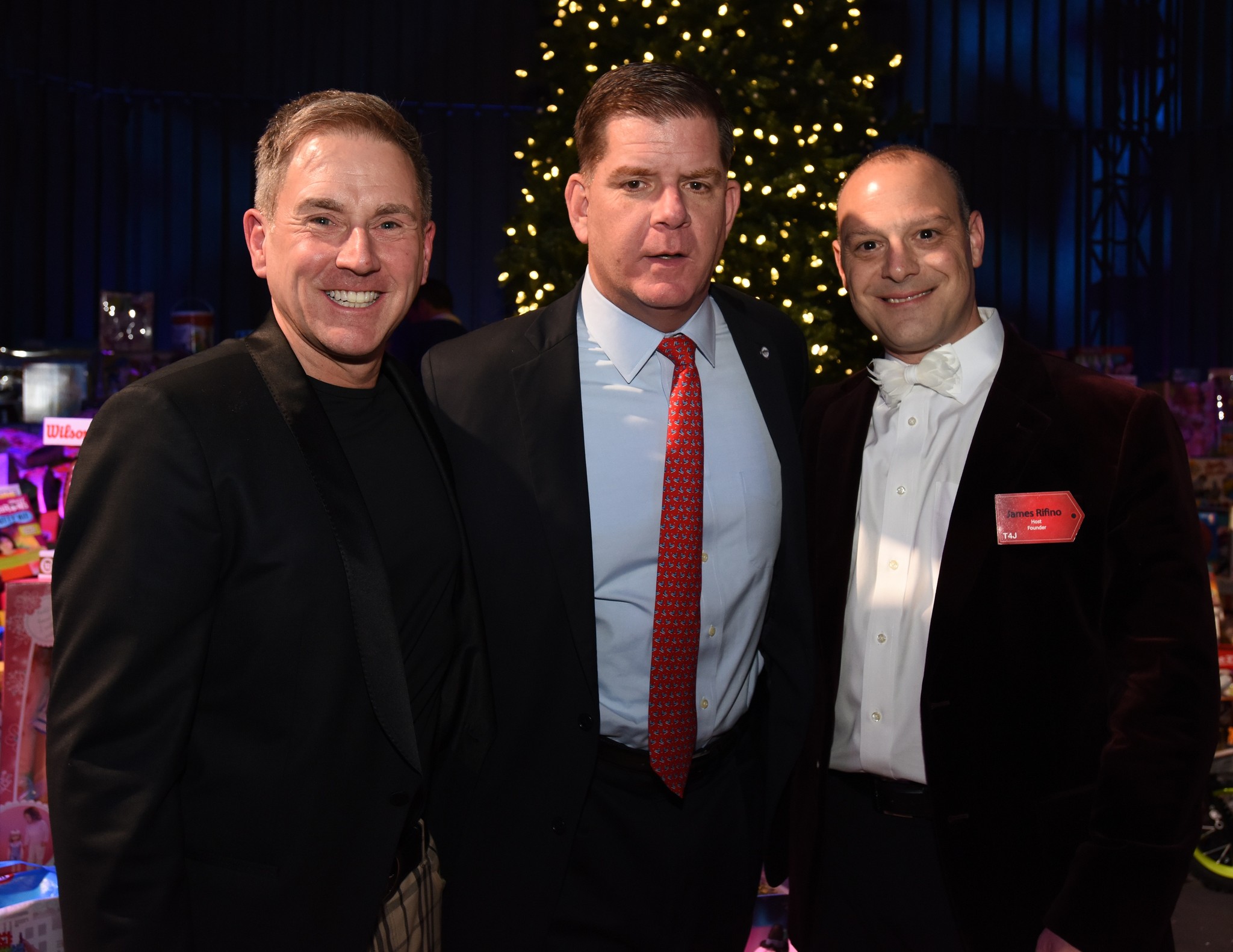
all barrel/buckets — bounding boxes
[173,310,214,358]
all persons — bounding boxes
[52,87,467,950]
[780,143,1216,952]
[411,63,814,951]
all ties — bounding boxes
[649,337,703,798]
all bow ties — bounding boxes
[867,343,963,408]
